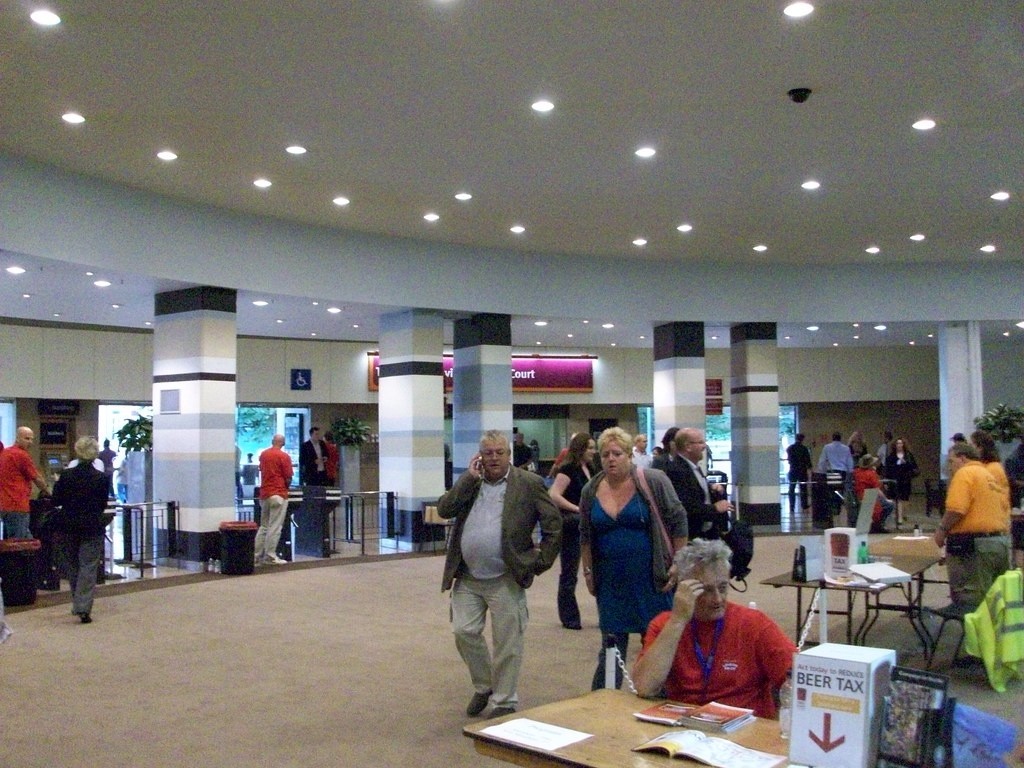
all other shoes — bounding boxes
[254,557,264,564]
[264,558,288,564]
[80,612,92,623]
[466,689,492,717]
[487,707,516,719]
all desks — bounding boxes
[463,687,788,768]
[758,531,946,658]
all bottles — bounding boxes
[913,525,920,536]
[858,542,868,563]
[1020,498,1024,512]
[779,670,792,739]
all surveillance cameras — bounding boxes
[787,88,813,104]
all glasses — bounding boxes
[689,440,705,444]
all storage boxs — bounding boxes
[824,526,857,579]
[788,643,896,768]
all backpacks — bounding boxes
[718,516,754,592]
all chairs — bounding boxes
[417,501,456,557]
[926,566,1024,671]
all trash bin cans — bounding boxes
[2,538,42,605]
[925,478,946,520]
[216,521,258,575]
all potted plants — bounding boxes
[112,413,154,559]
[331,415,371,533]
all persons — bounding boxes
[253,433,295,568]
[297,427,329,486]
[785,430,922,534]
[1,426,51,539]
[437,430,564,722]
[934,428,1024,671]
[627,534,800,726]
[527,438,541,473]
[512,431,532,471]
[547,426,736,692]
[234,445,244,504]
[322,430,340,488]
[48,434,121,624]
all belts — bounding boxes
[951,532,1001,538]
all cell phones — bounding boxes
[478,456,483,470]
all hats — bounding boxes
[858,454,879,468]
